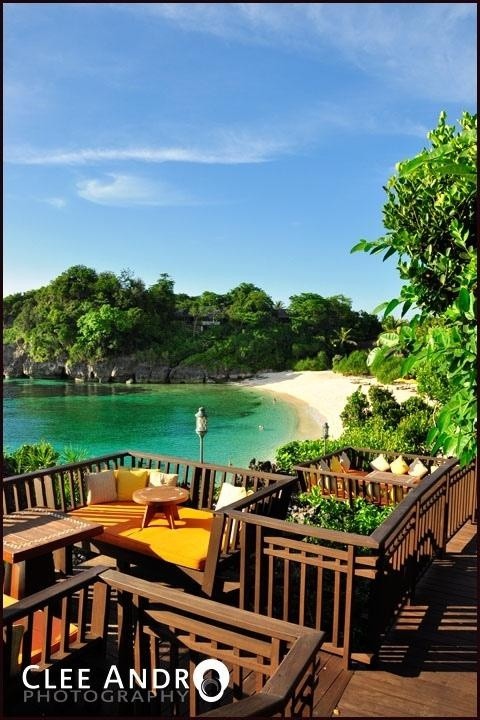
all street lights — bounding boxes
[195,405,208,464]
[320,422,329,457]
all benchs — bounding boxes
[66,486,247,599]
[2,593,78,668]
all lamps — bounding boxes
[321,422,329,455]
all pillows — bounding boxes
[304,445,439,498]
[84,464,178,504]
[246,489,255,498]
[218,481,246,530]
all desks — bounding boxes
[1,504,105,606]
[130,486,192,531]
